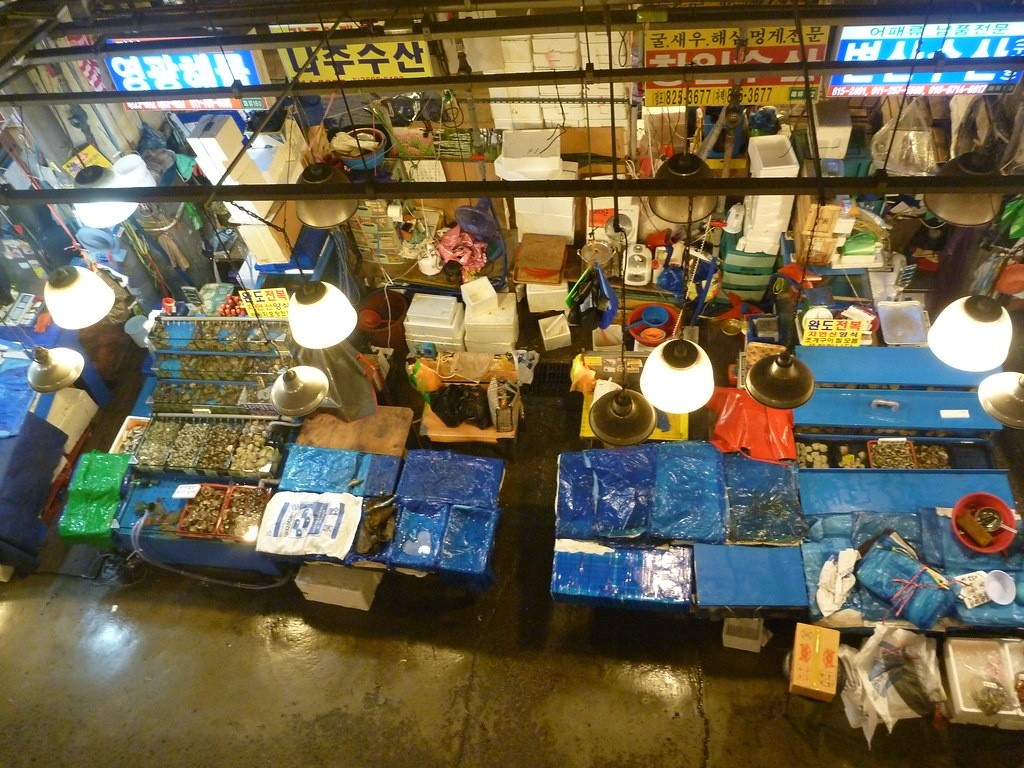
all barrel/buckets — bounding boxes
[357,291,407,349]
[124,315,151,348]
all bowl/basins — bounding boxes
[719,318,741,336]
[984,569,1016,605]
[640,328,666,341]
[334,126,387,170]
[951,493,1017,553]
[627,303,682,347]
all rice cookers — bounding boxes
[623,243,652,286]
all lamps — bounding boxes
[587,226,657,449]
[199,205,329,417]
[281,228,358,349]
[744,202,822,410]
[0,200,115,331]
[927,245,1023,373]
[978,371,1023,428]
[0,305,85,391]
[648,86,719,224]
[34,63,139,226]
[924,71,1014,228]
[639,197,715,413]
[294,96,359,227]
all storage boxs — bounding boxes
[43,387,99,482]
[294,564,384,610]
[187,112,884,355]
[942,636,1024,729]
[789,623,841,703]
[867,441,917,471]
[719,616,765,653]
[110,318,292,545]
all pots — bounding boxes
[576,240,616,269]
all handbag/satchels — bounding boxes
[487,375,520,432]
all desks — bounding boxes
[581,383,688,446]
[419,399,523,465]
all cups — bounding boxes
[417,244,443,276]
